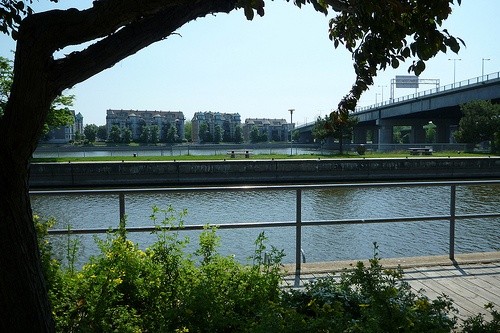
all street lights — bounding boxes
[288,108,294,157]
[377,85,388,106]
[482,58,491,81]
[448,58,462,89]
[376,93,381,107]
